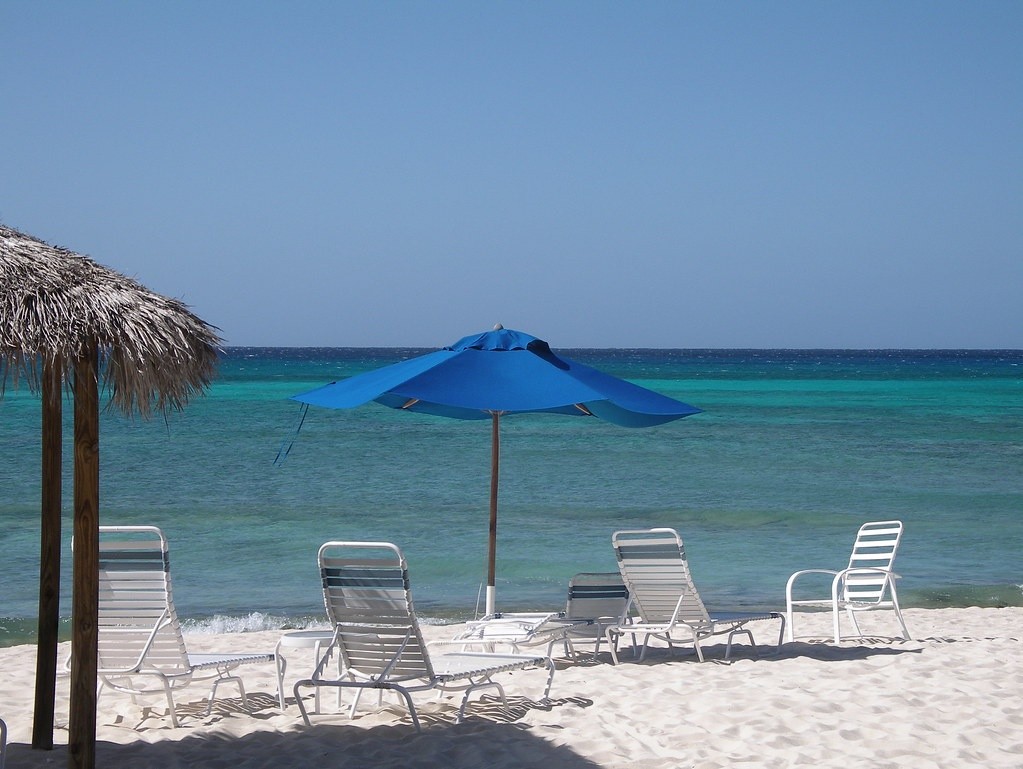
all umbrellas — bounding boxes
[271,323,707,616]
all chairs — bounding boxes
[56,525,287,728]
[606,528,785,665]
[786,520,912,646]
[293,541,554,736]
[426,573,632,671]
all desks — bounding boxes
[274,630,344,713]
